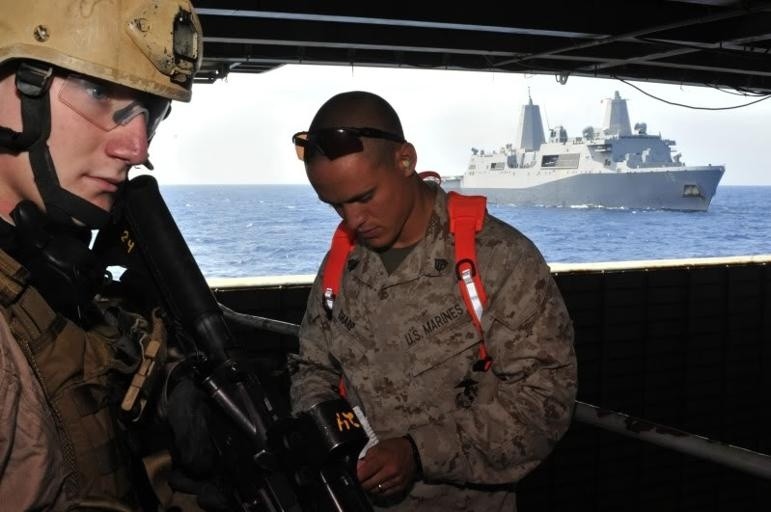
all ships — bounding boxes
[452,81,726,215]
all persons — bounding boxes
[2,0,204,512]
[287,91,580,511]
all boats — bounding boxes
[427,172,464,195]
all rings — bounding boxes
[378,482,385,492]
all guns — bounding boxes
[90,173,352,512]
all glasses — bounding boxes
[292,127,405,161]
[59,72,171,141]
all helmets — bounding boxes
[0,1,204,103]
